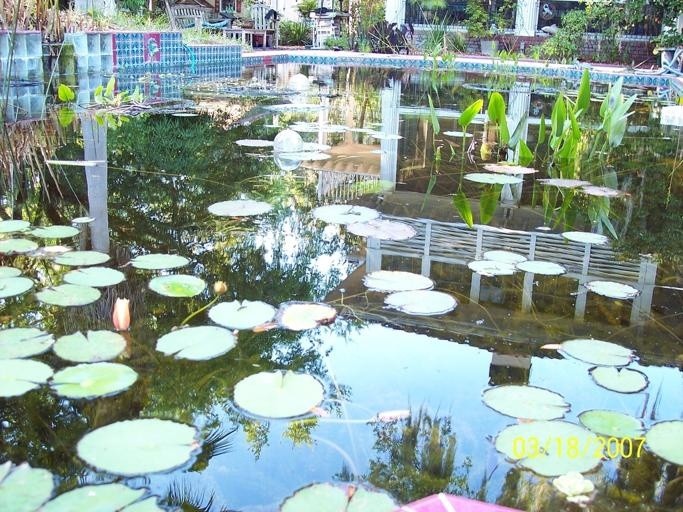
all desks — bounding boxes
[221,27,275,49]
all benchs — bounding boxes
[171,3,232,39]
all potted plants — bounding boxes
[236,15,255,28]
[463,0,528,55]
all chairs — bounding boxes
[245,5,281,48]
